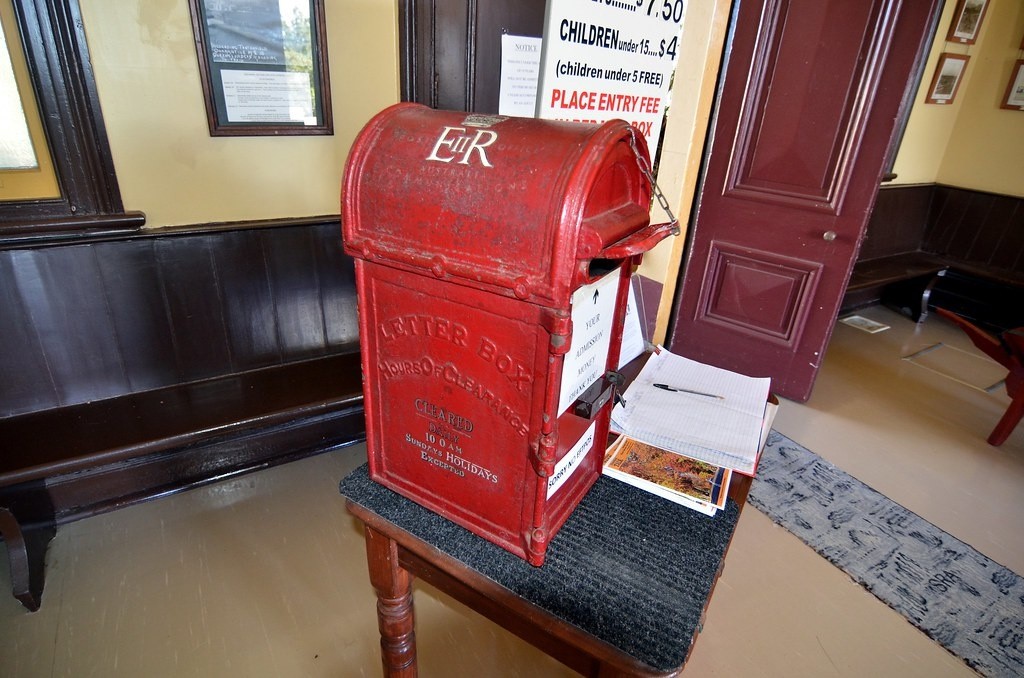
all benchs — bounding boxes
[1,214,366,612]
[847,251,953,323]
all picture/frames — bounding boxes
[189,0,334,136]
[925,52,971,104]
[945,0,990,45]
[1000,59,1024,111]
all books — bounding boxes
[609,344,771,478]
[602,435,732,517]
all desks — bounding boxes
[339,395,777,678]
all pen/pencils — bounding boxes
[653,384,725,399]
